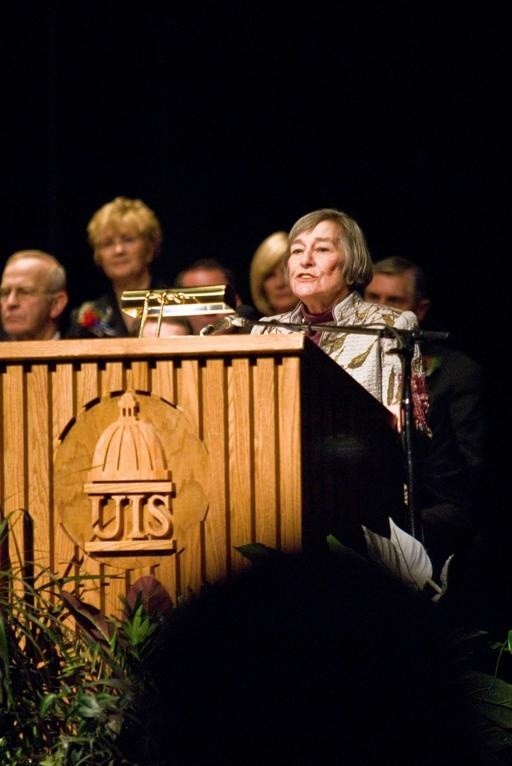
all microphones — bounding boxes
[199,304,256,335]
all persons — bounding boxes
[114,543,487,766]
